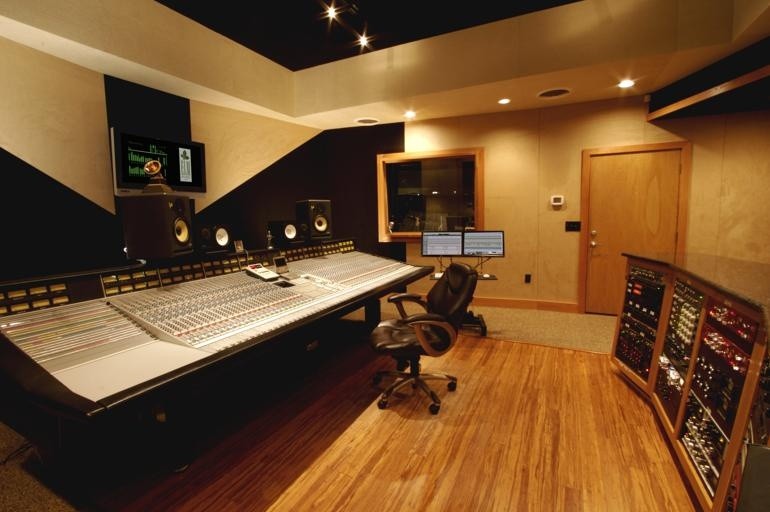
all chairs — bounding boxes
[371,264,478,417]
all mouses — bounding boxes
[483,273,490,278]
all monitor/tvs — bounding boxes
[421,230,462,256]
[462,230,505,257]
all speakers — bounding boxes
[295,198,333,243]
[119,194,195,267]
[198,222,232,259]
[266,219,305,249]
